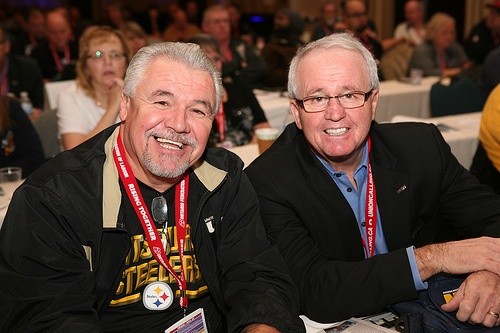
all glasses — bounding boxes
[151,195,168,255]
[293,87,376,113]
[87,49,125,61]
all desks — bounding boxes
[0,112,483,232]
[373,73,440,122]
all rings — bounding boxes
[486,310,499,320]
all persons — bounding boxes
[0,0,500,178]
[470,82,500,193]
[0,42,303,333]
[244,33,500,332]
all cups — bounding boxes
[0,167,22,203]
[256,127,280,156]
[411,69,423,84]
